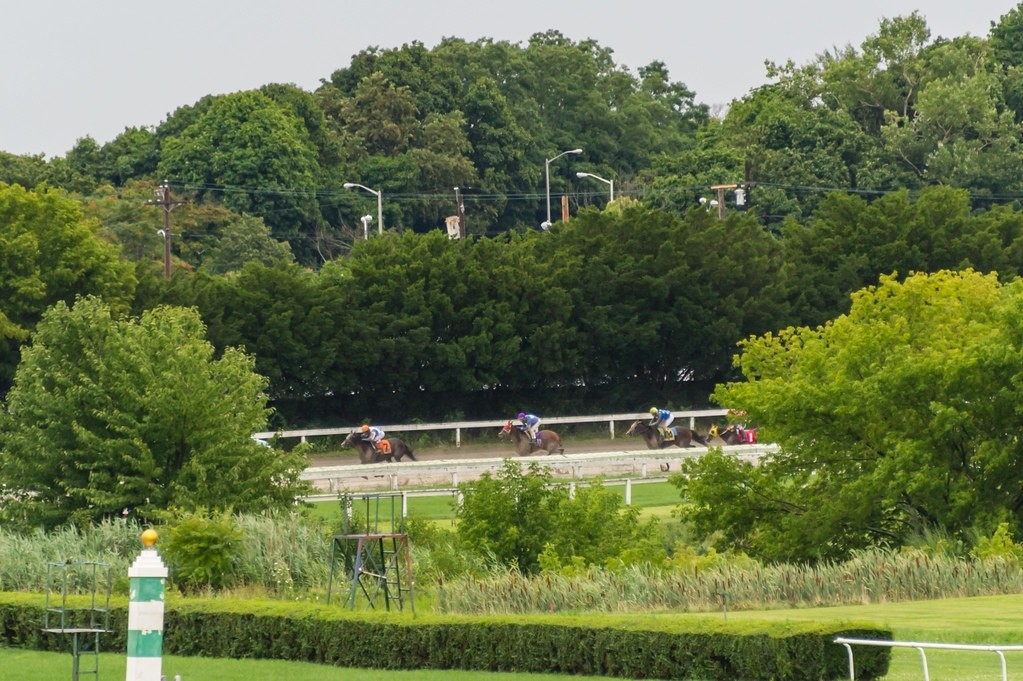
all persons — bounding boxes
[648,407,675,438]
[517,412,543,445]
[360,424,386,454]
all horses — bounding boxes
[706,423,757,445]
[498,420,564,456]
[341,430,418,464]
[625,419,709,450]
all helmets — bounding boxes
[518,413,525,419]
[650,407,658,413]
[362,425,369,433]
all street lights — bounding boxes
[157,229,170,278]
[576,171,613,200]
[734,184,750,214]
[360,214,373,241]
[454,187,460,203]
[343,182,383,236]
[545,148,583,228]
